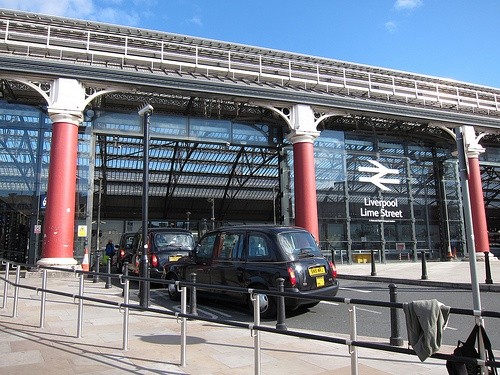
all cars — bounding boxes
[115,224,195,289]
[164,221,340,320]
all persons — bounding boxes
[105,238,115,267]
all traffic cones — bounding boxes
[445,244,454,257]
[81,247,90,272]
[452,247,457,260]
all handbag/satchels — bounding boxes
[445,324,498,375]
